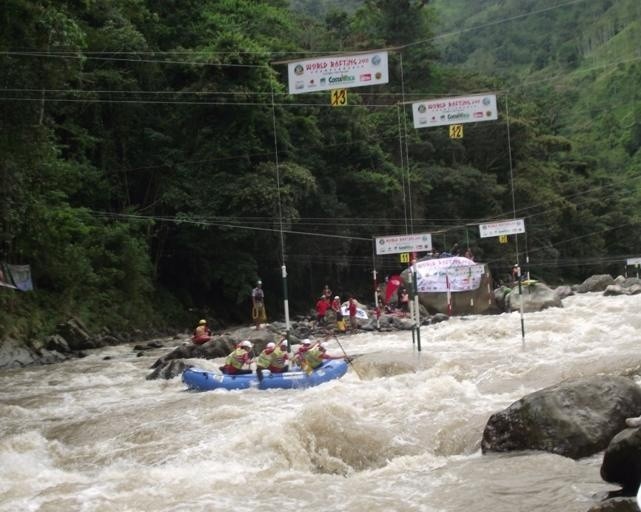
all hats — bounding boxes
[256,281,262,284]
[334,296,340,299]
[320,295,325,299]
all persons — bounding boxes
[271,338,293,373]
[321,284,333,297]
[316,294,331,325]
[334,305,347,333]
[512,261,529,284]
[305,331,353,372]
[332,296,348,333]
[295,338,321,370]
[194,319,213,337]
[221,340,256,375]
[256,336,289,376]
[374,287,385,314]
[345,298,359,330]
[251,280,269,330]
[425,242,477,262]
[399,288,409,313]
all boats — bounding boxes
[183,355,350,393]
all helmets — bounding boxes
[241,341,253,347]
[267,343,275,347]
[282,339,287,346]
[303,339,310,344]
[199,319,206,323]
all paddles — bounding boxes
[253,348,264,381]
[294,353,313,376]
